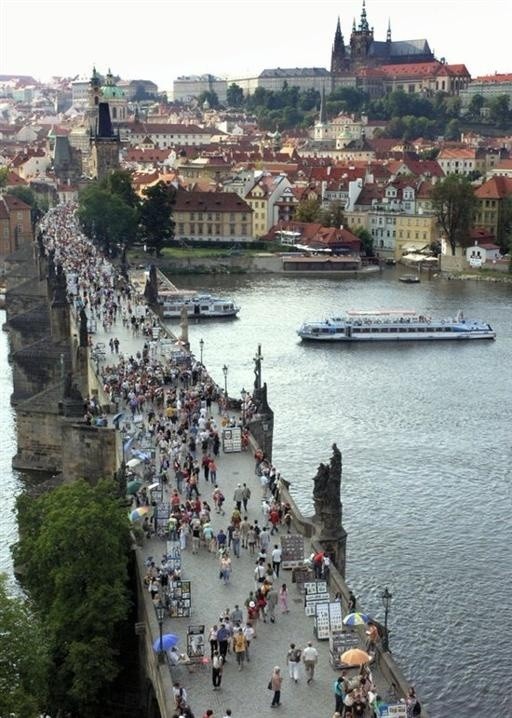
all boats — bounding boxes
[157,290,243,318]
[385,257,397,266]
[296,310,500,343]
[398,271,421,284]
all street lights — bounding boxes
[260,417,270,459]
[221,363,229,395]
[152,598,168,662]
[240,386,247,425]
[199,338,204,365]
[380,585,393,652]
